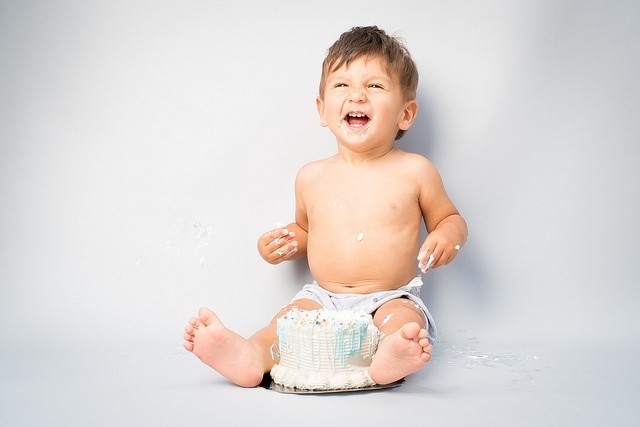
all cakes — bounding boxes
[271,308,382,391]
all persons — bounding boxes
[183,26,468,388]
